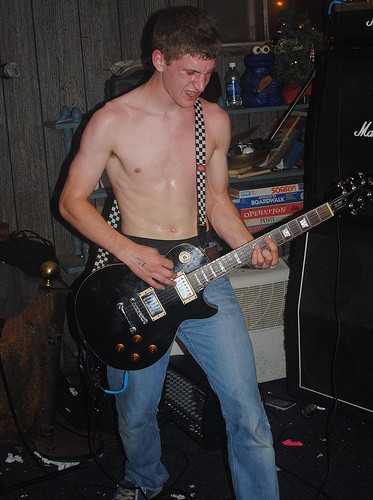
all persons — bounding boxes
[58,6,279,500]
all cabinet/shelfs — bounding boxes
[221,41,311,183]
[44,113,113,281]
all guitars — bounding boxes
[67,170,372,372]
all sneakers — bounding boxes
[114,481,163,499]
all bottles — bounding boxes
[225,61,242,109]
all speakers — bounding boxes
[262,390,317,476]
